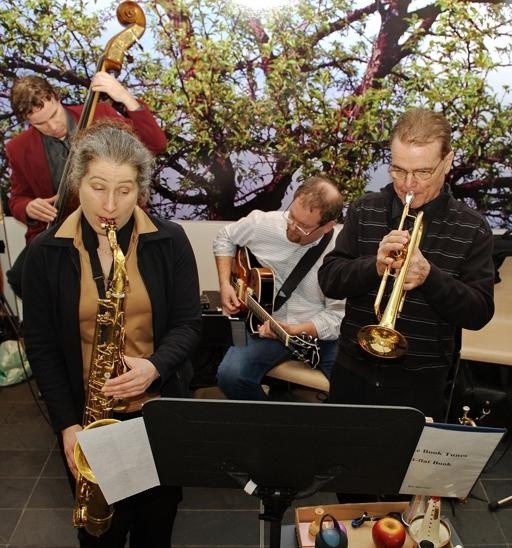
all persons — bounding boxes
[213,176,348,482]
[316,104,499,504]
[22,126,204,546]
[2,70,166,245]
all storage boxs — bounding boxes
[294,501,454,548]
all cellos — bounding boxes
[39,0,147,233]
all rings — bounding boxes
[384,256,389,264]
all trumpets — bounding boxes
[357,190,426,359]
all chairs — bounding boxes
[266,357,331,403]
[444,255,512,426]
[168,220,239,292]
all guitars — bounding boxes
[215,245,322,370]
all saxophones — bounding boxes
[72,218,142,538]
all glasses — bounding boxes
[388,157,444,181]
[281,199,336,237]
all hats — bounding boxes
[0,340,32,386]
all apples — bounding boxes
[371,517,407,548]
